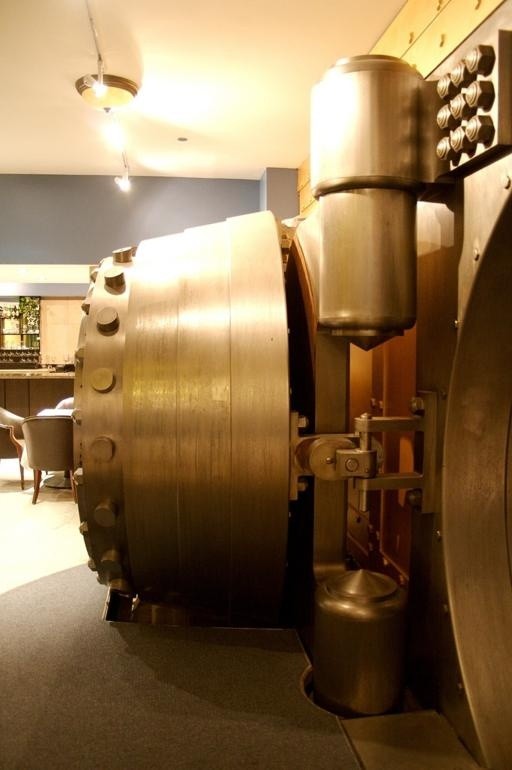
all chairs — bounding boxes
[0,398,75,504]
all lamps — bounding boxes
[75,75,139,113]
[84,0,130,192]
